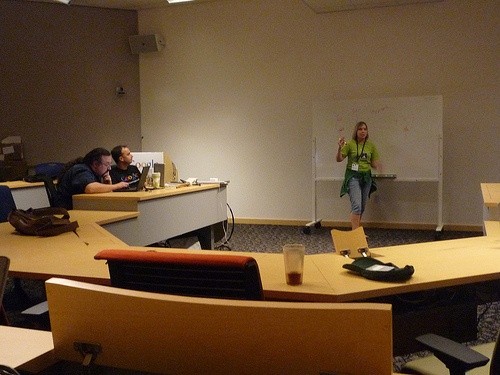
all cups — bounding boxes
[152,173,160,188]
[283,243,305,285]
[145,166,154,187]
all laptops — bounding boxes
[115,166,150,192]
[154,163,165,187]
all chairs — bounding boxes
[0,163,265,375]
[400,331,500,375]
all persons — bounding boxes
[52,147,129,210]
[336,121,380,239]
[109,145,141,192]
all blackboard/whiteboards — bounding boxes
[312,95,444,181]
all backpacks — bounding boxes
[9,207,79,237]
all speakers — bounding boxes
[129,33,163,54]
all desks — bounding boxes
[0,183,500,375]
